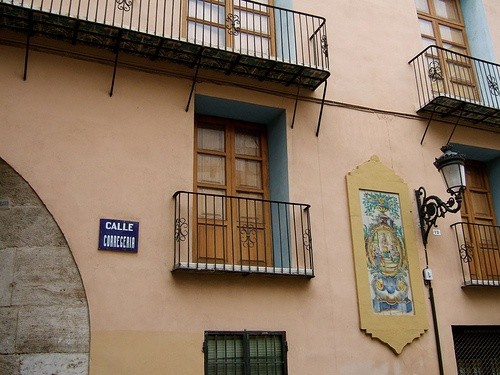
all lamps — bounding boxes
[414,146,467,245]
